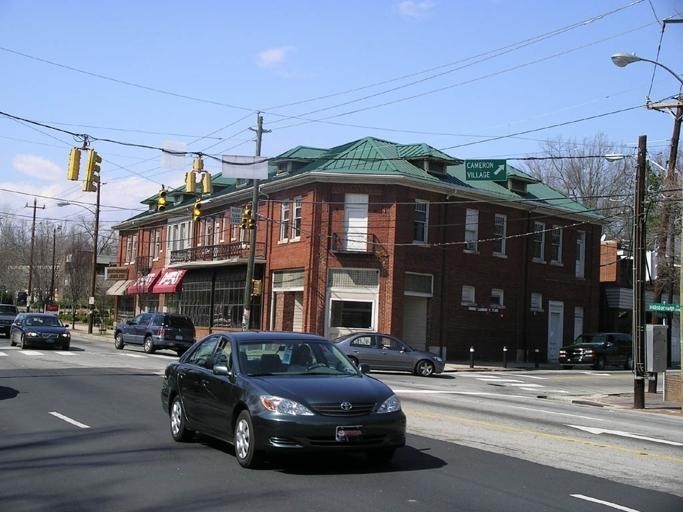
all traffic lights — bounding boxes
[252,280,261,295]
[244,206,250,217]
[192,202,202,220]
[241,217,248,230]
[156,190,168,212]
[81,150,101,193]
[248,219,254,228]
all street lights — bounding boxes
[57,202,98,336]
[608,51,683,89]
[603,153,669,180]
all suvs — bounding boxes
[114,312,195,358]
[0,303,18,338]
[558,330,634,370]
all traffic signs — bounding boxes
[464,159,506,182]
[648,302,680,313]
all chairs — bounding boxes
[228,352,284,373]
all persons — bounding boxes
[282,345,315,373]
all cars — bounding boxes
[8,312,70,351]
[317,331,445,377]
[160,329,405,468]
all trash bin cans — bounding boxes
[429,346,446,362]
